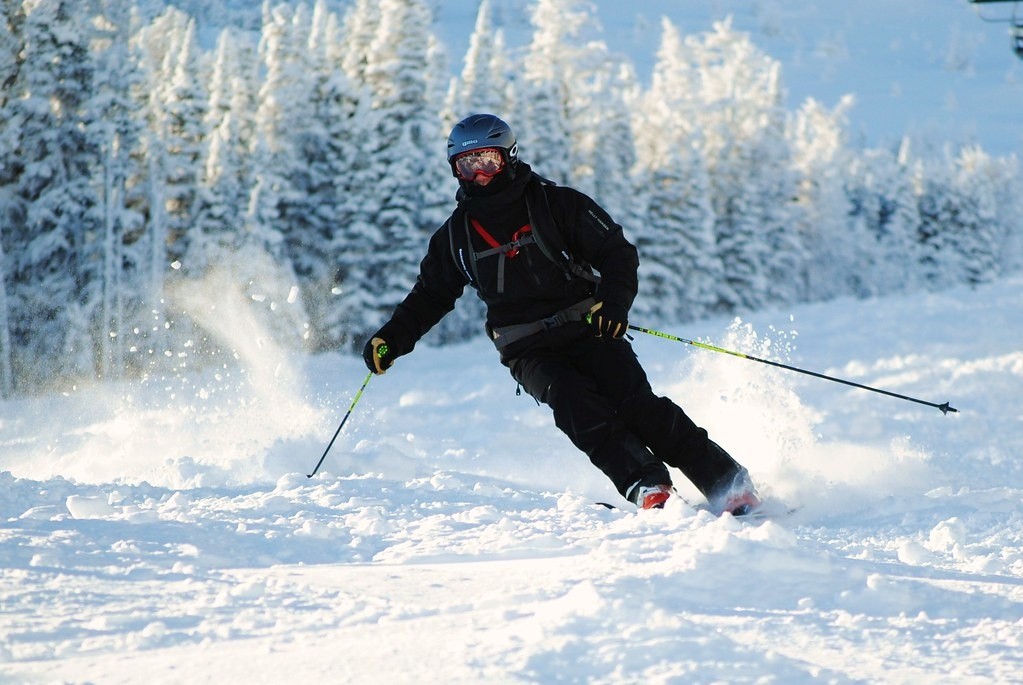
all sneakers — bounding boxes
[637,484,673,512]
[725,487,762,513]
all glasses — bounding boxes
[452,147,506,181]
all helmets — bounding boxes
[447,113,518,185]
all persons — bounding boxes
[361,114,759,517]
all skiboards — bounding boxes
[592,482,802,522]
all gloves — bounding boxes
[363,337,394,375]
[588,298,630,340]
